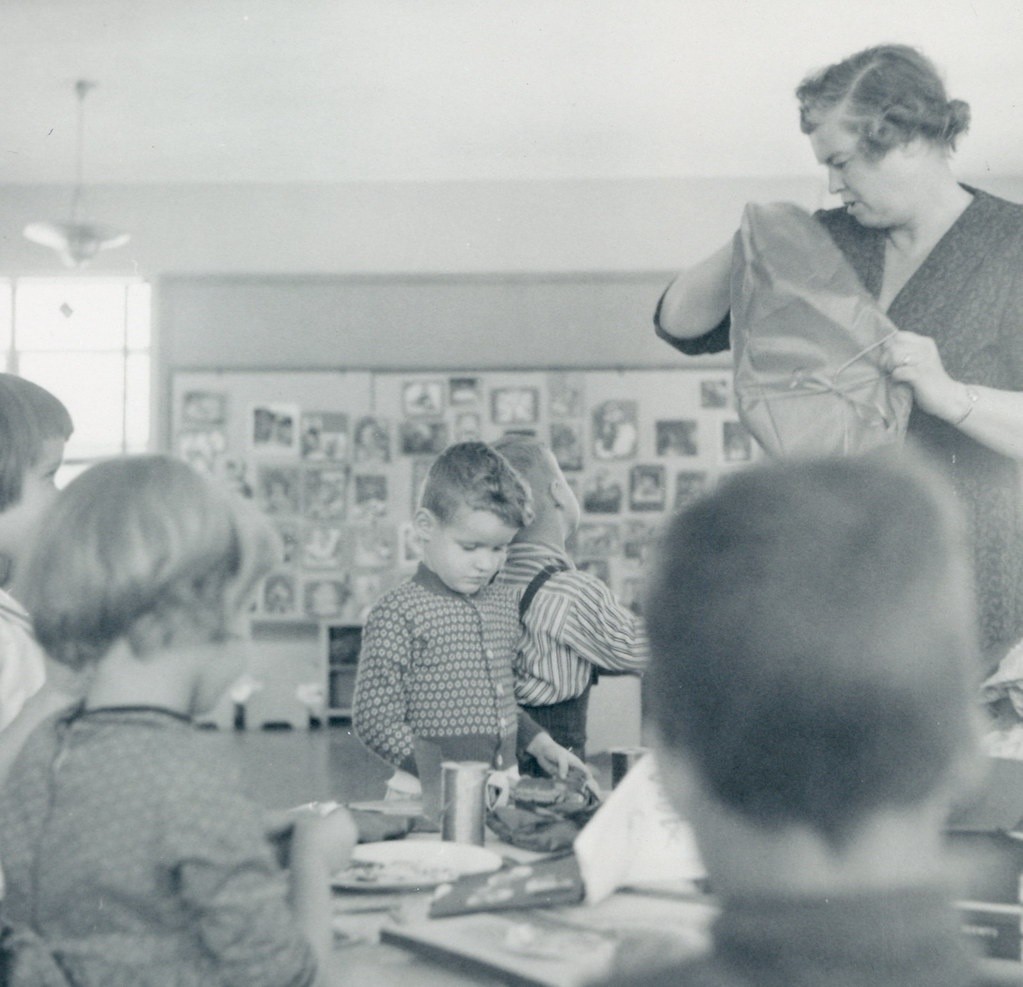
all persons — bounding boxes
[492,433,649,781]
[352,442,595,799]
[0,374,73,775]
[179,379,752,623]
[572,455,1023,985]
[0,454,357,987]
[653,43,1023,669]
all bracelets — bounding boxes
[956,385,984,424]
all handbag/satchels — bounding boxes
[487,770,605,854]
[730,198,913,464]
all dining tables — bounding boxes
[267,791,724,987]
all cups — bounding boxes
[441,759,490,847]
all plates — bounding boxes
[332,839,503,892]
